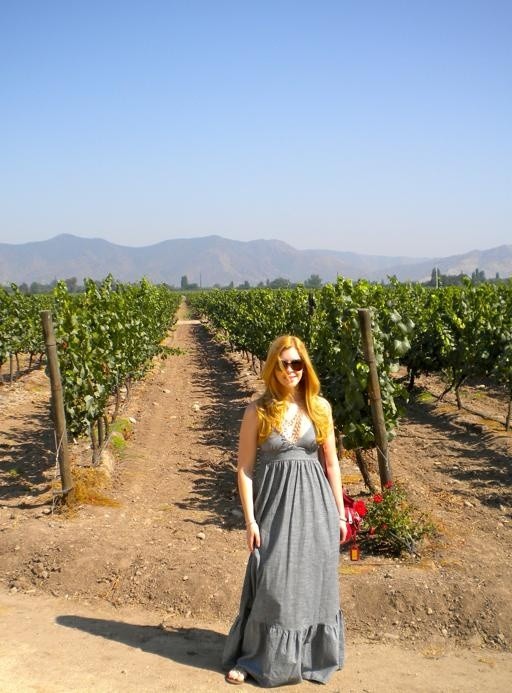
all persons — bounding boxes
[226,334,350,687]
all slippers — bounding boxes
[225,668,247,684]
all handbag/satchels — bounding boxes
[340,493,354,544]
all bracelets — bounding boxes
[245,518,260,528]
[338,514,349,523]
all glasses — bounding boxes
[275,360,303,371]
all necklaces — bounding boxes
[269,398,312,446]
